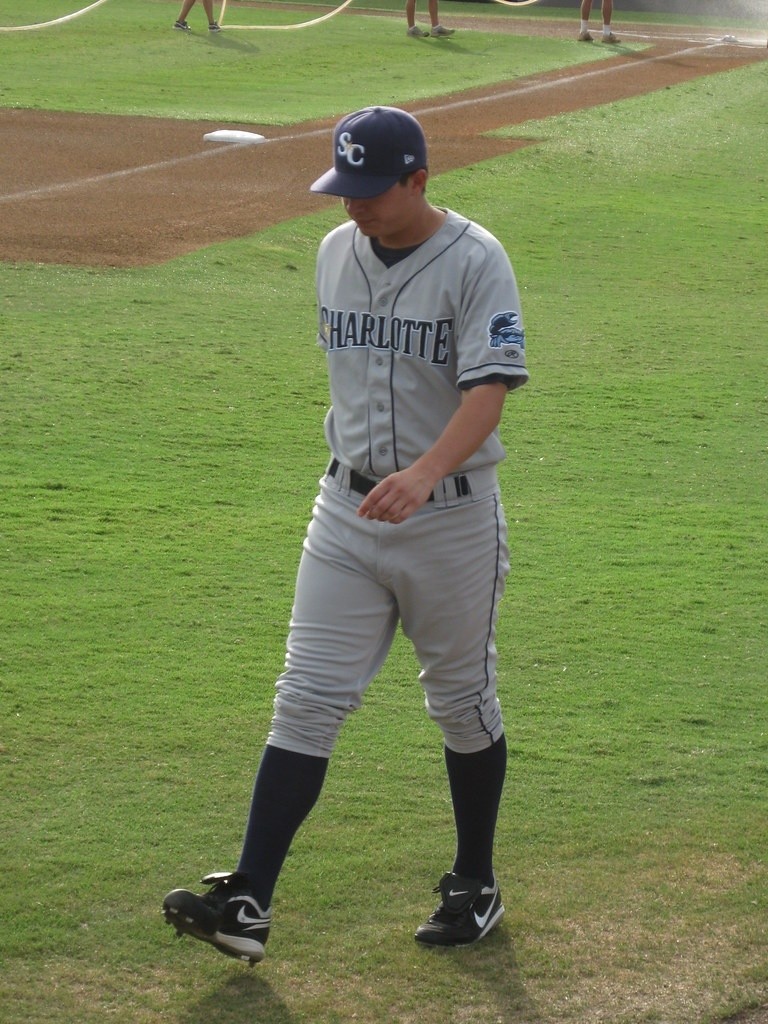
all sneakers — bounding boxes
[407,26,429,38]
[431,26,454,38]
[415,870,504,948]
[163,873,274,963]
[209,22,221,32]
[172,21,191,31]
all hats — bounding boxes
[311,106,429,199]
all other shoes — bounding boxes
[602,32,620,43]
[577,32,592,41]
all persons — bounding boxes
[162,106,529,969]
[173,0,222,31]
[406,0,455,37]
[578,0,620,43]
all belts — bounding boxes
[330,460,469,501]
